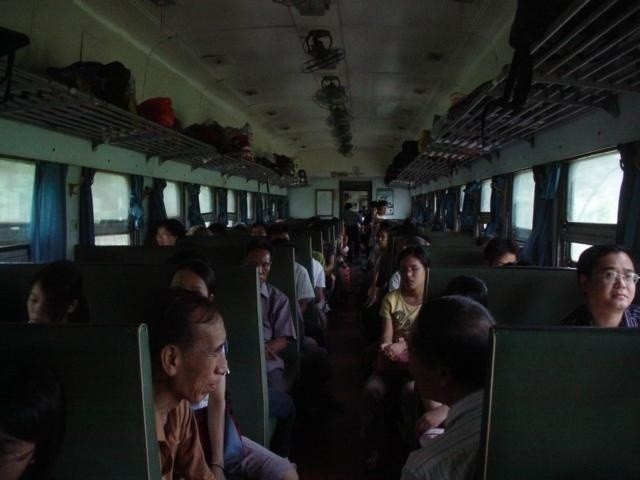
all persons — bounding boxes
[248,218,431,470]
[3,359,66,480]
[28,260,86,324]
[402,276,496,480]
[476,234,530,267]
[562,245,639,327]
[138,260,300,480]
[154,219,245,245]
[342,202,386,265]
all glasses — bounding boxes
[602,270,639,285]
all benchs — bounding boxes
[390,219,640,480]
[1,219,345,480]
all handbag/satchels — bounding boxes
[185,118,253,161]
[498,0,572,111]
[384,141,419,184]
[420,81,495,154]
[257,153,310,187]
[56,60,175,129]
